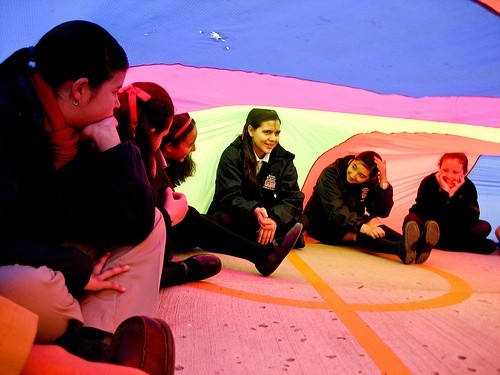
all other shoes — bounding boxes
[104,315,175,375]
[398,221,420,264]
[417,221,440,264]
[254,222,303,277]
[169,254,222,284]
[293,233,305,249]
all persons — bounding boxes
[302,150,440,264]
[402,152,493,248]
[495,226,500,241]
[0,20,301,375]
